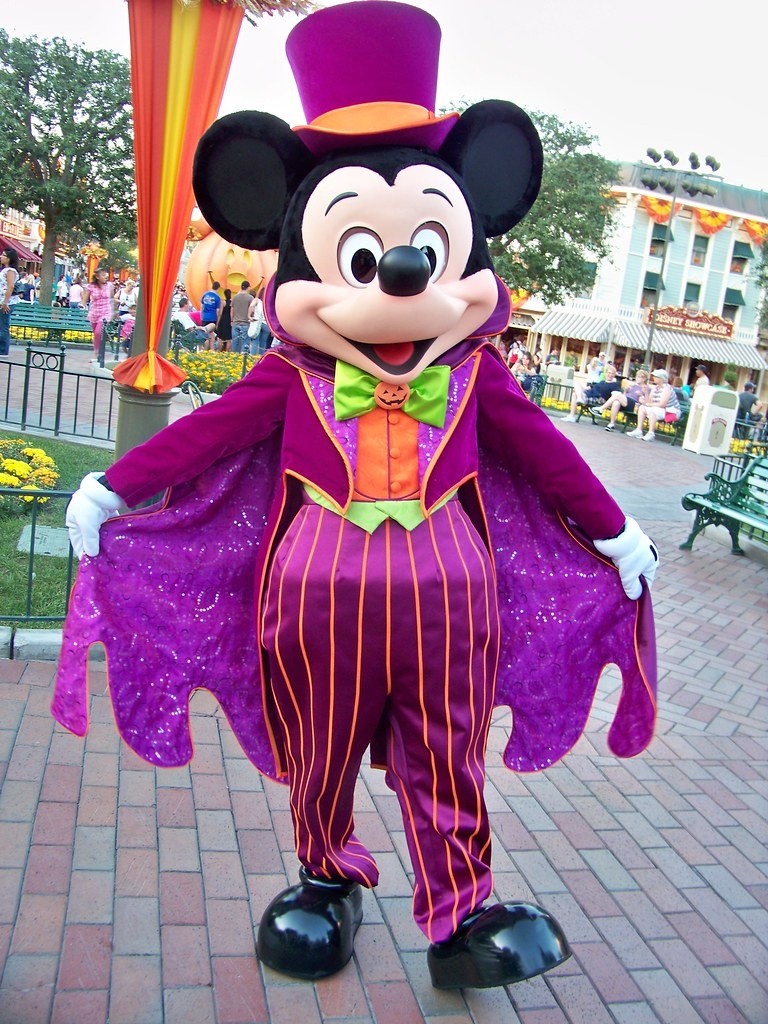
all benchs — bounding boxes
[678,455,768,557]
[576,380,690,446]
[172,320,199,353]
[732,414,755,440]
[6,301,123,353]
[513,374,550,409]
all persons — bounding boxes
[588,369,651,432]
[560,367,620,422]
[499,340,763,421]
[0,248,20,357]
[14,269,275,364]
[626,369,681,441]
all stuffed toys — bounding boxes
[49,0,660,991]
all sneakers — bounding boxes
[626,429,642,438]
[604,424,614,432]
[560,417,575,423]
[641,432,655,441]
[588,407,602,416]
[576,399,584,406]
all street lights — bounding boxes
[640,147,721,372]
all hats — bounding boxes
[744,382,757,387]
[242,281,251,287]
[695,364,706,373]
[651,369,667,379]
[181,288,186,291]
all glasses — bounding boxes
[2,254,7,256]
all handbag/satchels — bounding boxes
[248,321,262,339]
[11,281,26,295]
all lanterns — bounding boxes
[183,217,278,312]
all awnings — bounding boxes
[652,223,674,242]
[733,240,755,259]
[643,271,666,291]
[531,310,612,343]
[0,236,43,264]
[724,287,746,306]
[613,319,768,370]
